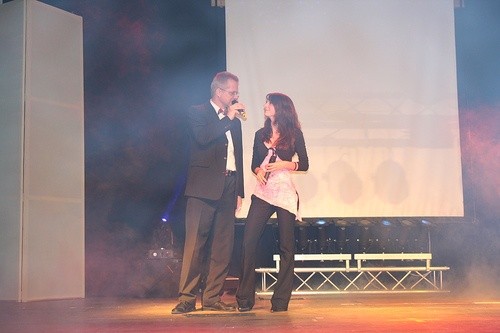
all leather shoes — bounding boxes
[270,304,288,312]
[238,304,252,311]
[171,301,196,313]
[202,299,236,311]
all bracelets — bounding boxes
[291,162,297,172]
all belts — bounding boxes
[225,170,237,177]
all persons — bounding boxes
[236,93,310,311]
[171,72,245,314]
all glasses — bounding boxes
[217,86,239,95]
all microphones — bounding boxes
[232,100,247,120]
[264,156,276,186]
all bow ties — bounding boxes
[217,108,228,115]
